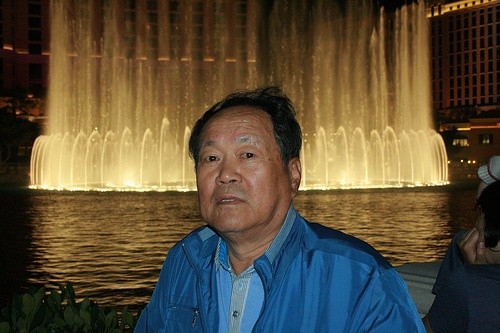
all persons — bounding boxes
[422,156,500,333]
[134,86,427,333]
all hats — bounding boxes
[478,155,500,184]
